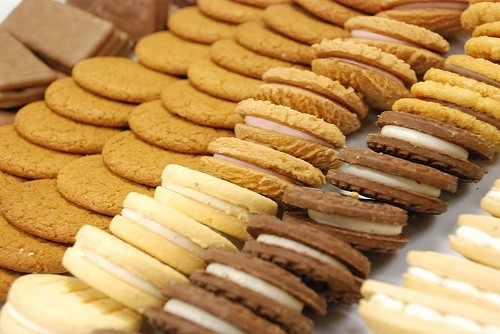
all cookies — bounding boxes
[1,0,500,333]
